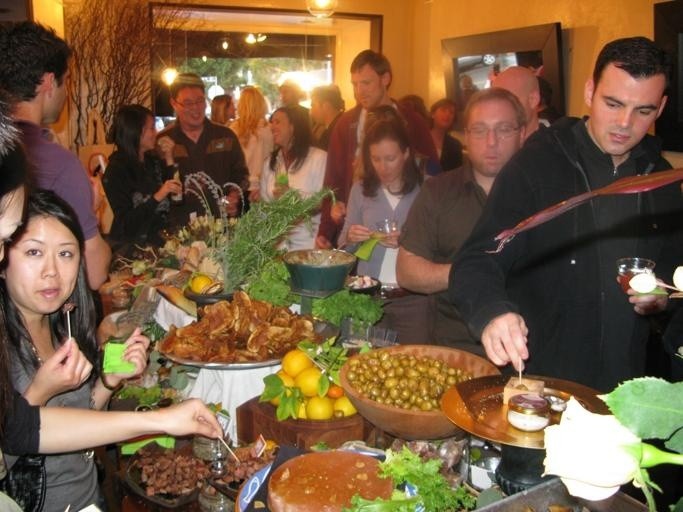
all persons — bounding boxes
[395,86,528,356]
[448,36,682,394]
[0,19,113,291]
[491,66,542,140]
[535,76,560,125]
[0,187,150,512]
[102,49,478,346]
[0,107,225,480]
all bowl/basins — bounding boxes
[283,249,356,290]
[352,275,381,296]
[339,343,506,439]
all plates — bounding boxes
[165,321,338,370]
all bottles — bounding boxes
[171,163,184,204]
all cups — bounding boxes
[618,258,655,293]
[377,220,398,232]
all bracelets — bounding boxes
[100,372,118,391]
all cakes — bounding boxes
[265,449,394,512]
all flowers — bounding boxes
[539,375,683,512]
[628,265,683,298]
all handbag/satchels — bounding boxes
[0,454,46,512]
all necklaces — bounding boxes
[30,341,44,366]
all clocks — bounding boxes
[481,53,496,66]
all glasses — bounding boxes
[464,125,522,137]
[172,97,206,106]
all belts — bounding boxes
[378,288,409,299]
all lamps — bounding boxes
[243,32,268,46]
[303,0,339,19]
